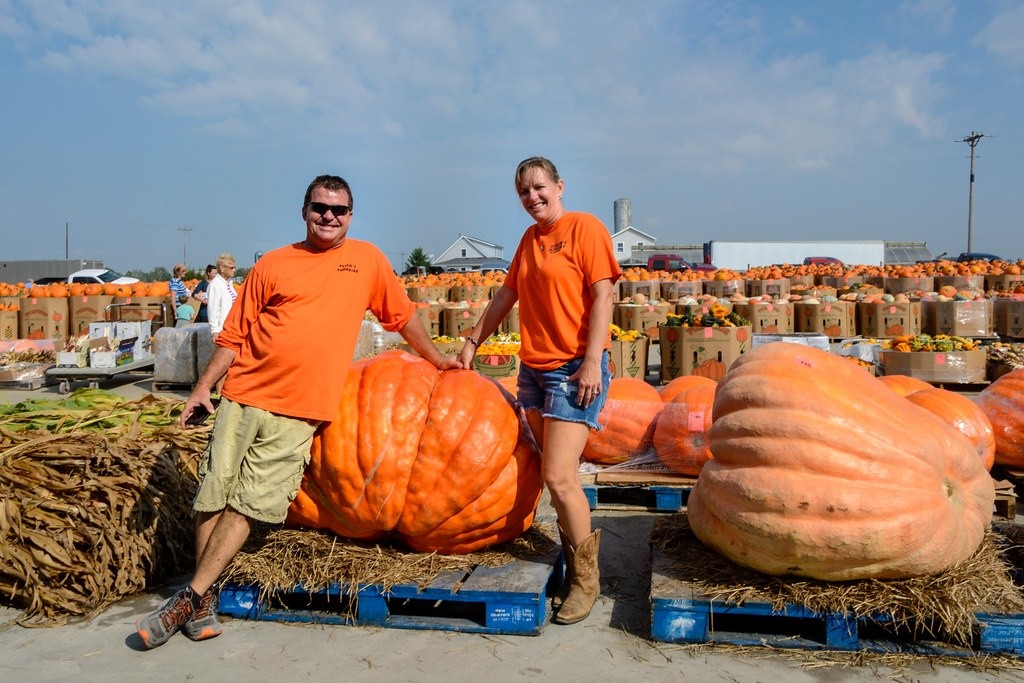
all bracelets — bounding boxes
[468,335,479,348]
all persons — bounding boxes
[169,255,239,400]
[137,174,465,649]
[454,157,622,625]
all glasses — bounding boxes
[224,267,235,270]
[306,202,352,216]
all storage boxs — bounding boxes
[0,293,214,382]
[607,274,1024,399]
[351,284,521,382]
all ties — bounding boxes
[227,284,236,303]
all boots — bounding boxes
[552,521,565,607]
[554,529,601,624]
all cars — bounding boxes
[401,266,508,277]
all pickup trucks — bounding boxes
[33,268,140,285]
[619,253,719,275]
[772,256,844,269]
[915,253,1002,264]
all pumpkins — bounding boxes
[610,300,750,340]
[395,270,506,308]
[401,330,522,355]
[581,341,1024,582]
[0,279,242,311]
[617,259,1024,306]
[285,348,544,555]
[839,334,981,367]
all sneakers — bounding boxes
[139,588,194,650]
[184,590,222,641]
